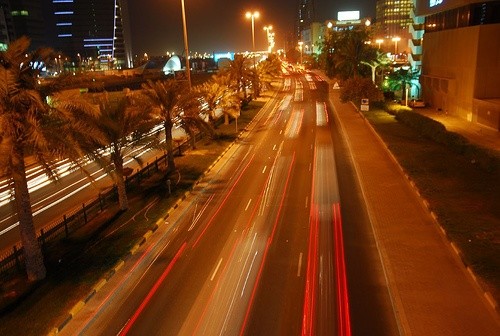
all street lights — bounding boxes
[247,12,259,99]
[392,38,400,55]
[263,25,275,52]
[298,41,304,63]
[376,39,382,49]
